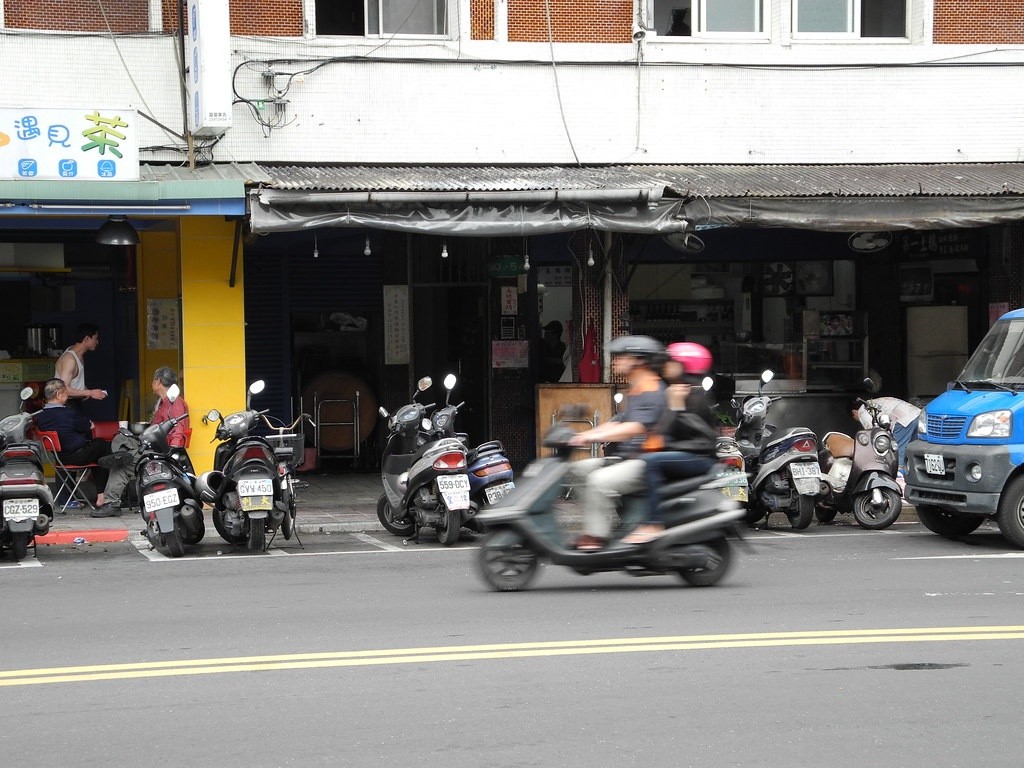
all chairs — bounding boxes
[126,428,193,513]
[35,426,99,514]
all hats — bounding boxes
[543,321,563,334]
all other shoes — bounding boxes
[569,536,602,548]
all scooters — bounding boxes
[702,369,903,530]
[0,387,54,559]
[118,383,205,557]
[377,373,517,547]
[475,414,749,591]
[202,379,286,553]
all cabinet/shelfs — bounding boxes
[630,266,874,400]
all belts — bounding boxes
[67,448,83,459]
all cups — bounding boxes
[119,420,128,429]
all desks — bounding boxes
[536,381,618,467]
[297,370,375,469]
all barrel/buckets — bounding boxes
[25,323,46,358]
[43,322,64,358]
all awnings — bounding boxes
[138,160,1024,228]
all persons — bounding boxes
[32,379,110,509]
[54,322,108,441]
[541,320,567,381]
[566,335,717,550]
[88,367,190,517]
[852,396,920,499]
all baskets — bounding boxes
[269,432,306,465]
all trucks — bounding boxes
[904,308,1024,550]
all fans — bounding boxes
[847,231,893,254]
[661,231,705,254]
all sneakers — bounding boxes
[90,504,122,517]
[97,451,134,469]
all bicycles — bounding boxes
[257,414,316,549]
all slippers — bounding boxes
[61,502,83,508]
[619,529,666,542]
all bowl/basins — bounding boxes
[130,421,151,434]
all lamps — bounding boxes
[94,215,140,245]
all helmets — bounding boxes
[608,335,665,368]
[668,343,714,375]
